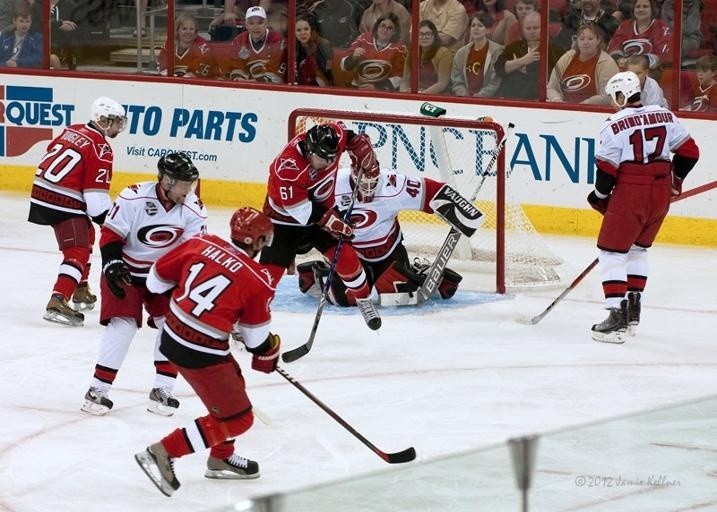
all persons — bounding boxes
[587,70,699,344]
[232,124,383,334]
[0,0,109,74]
[158,1,717,114]
[135,211,280,496]
[80,153,209,415]
[28,97,128,326]
[297,159,487,306]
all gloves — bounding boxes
[319,209,355,241]
[587,190,609,214]
[251,335,281,373]
[102,257,134,298]
[346,135,376,169]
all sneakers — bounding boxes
[354,295,381,330]
[85,387,113,408]
[150,388,178,408]
[207,453,257,474]
[73,287,97,303]
[147,442,181,489]
[592,291,640,332]
[47,293,84,320]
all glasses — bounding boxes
[418,32,434,40]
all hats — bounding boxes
[246,6,266,19]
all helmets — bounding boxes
[303,125,339,169]
[231,208,275,251]
[350,161,380,202]
[158,154,199,205]
[90,98,127,138]
[605,71,641,108]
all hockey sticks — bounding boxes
[395,124,515,308]
[281,164,364,363]
[510,254,600,324]
[229,327,415,463]
